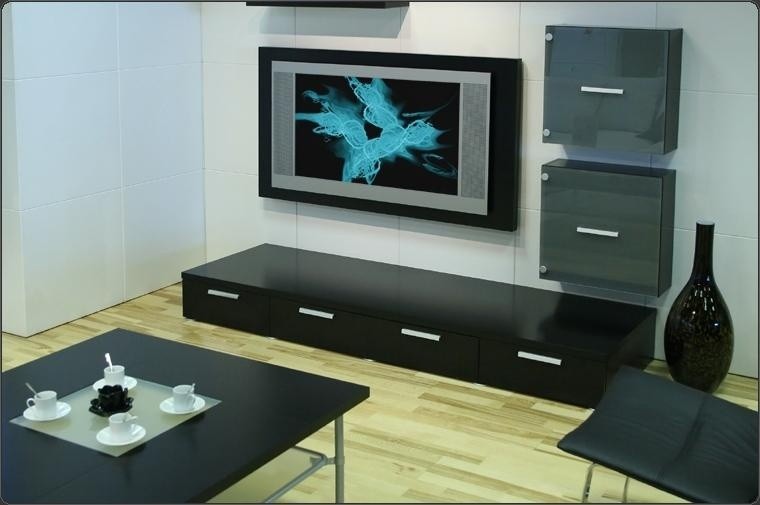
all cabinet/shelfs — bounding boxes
[180,243,658,411]
[539,159,676,296]
[542,24,684,155]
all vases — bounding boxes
[661,219,737,394]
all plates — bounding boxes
[22,400,72,422]
[89,395,135,417]
[95,425,147,446]
[92,376,138,392]
[158,395,206,417]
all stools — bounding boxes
[557,363,760,504]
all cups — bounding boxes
[25,391,59,412]
[171,384,196,409]
[107,413,140,436]
[103,365,126,385]
[97,385,129,409]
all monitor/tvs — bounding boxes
[257,45,522,232]
